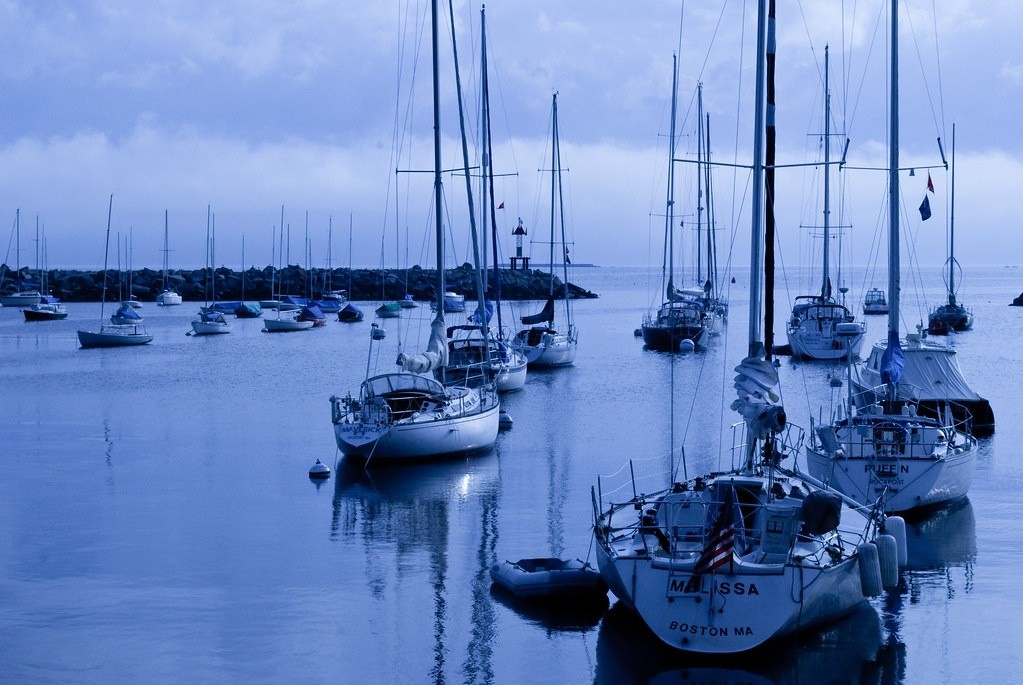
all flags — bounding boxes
[927,172,935,193]
[691,489,733,588]
[919,196,932,221]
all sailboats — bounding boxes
[76,194,152,349]
[110,231,145,326]
[263,204,316,333]
[189,1,996,659]
[396,227,417,309]
[374,235,405,318]
[0,208,60,307]
[336,213,365,322]
[157,207,183,305]
[22,215,68,320]
[190,204,236,333]
[430,223,466,313]
[122,228,144,308]
